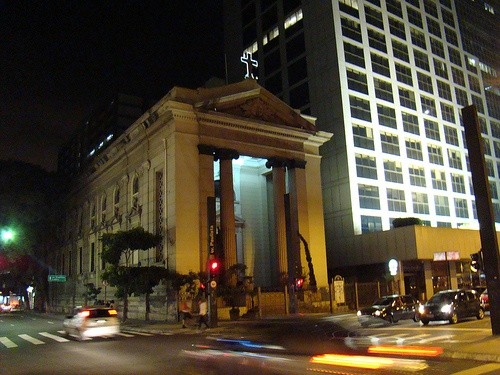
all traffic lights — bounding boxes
[293,275,304,292]
[209,260,219,271]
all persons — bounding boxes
[95,299,114,307]
[38,295,48,318]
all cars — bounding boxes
[62,308,121,342]
[479,289,490,311]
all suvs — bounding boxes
[419,289,484,324]
[356,294,422,328]
